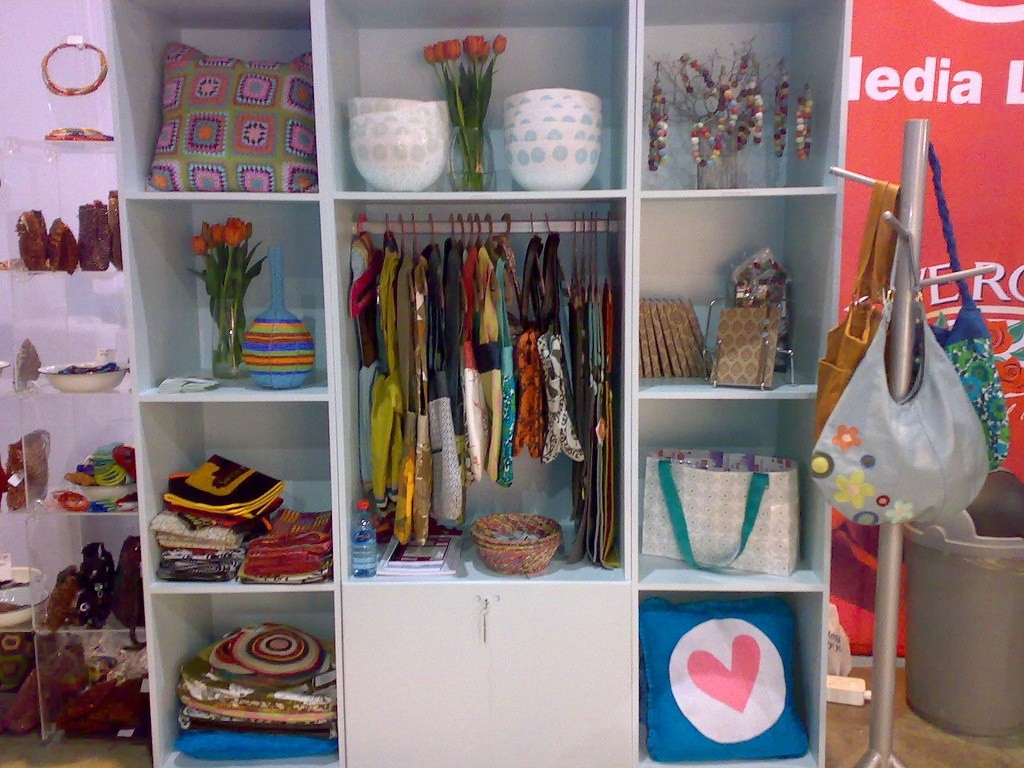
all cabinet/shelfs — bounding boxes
[633,0,854,768]
[318,1,637,768]
[106,0,346,768]
[0,137,151,744]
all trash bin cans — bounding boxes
[902,470,1024,738]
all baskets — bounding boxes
[470,513,563,576]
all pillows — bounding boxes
[176,731,336,761]
[639,596,808,763]
[146,41,319,192]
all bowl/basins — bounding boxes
[37,362,129,392]
[347,98,452,192]
[0,566,42,590]
[502,87,604,191]
[0,588,49,627]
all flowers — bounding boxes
[186,216,267,317]
[422,33,506,188]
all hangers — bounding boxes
[356,213,613,304]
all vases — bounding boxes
[697,123,734,187]
[446,127,493,191]
[211,298,245,379]
[245,245,314,389]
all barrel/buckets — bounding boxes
[905,470,1024,737]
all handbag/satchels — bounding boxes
[6,429,50,509]
[642,449,800,577]
[45,535,147,648]
[352,229,585,548]
[3,632,148,739]
[809,142,1010,525]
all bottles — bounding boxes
[352,500,377,578]
